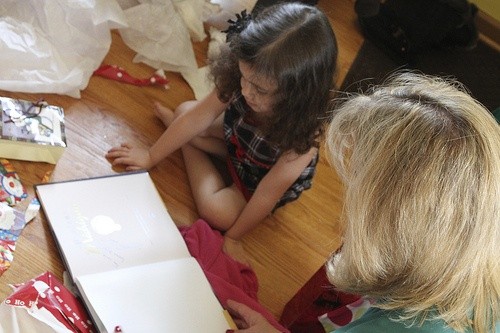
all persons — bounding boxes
[105,0,339,266]
[226,73,500,333]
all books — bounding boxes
[33,168,239,332]
